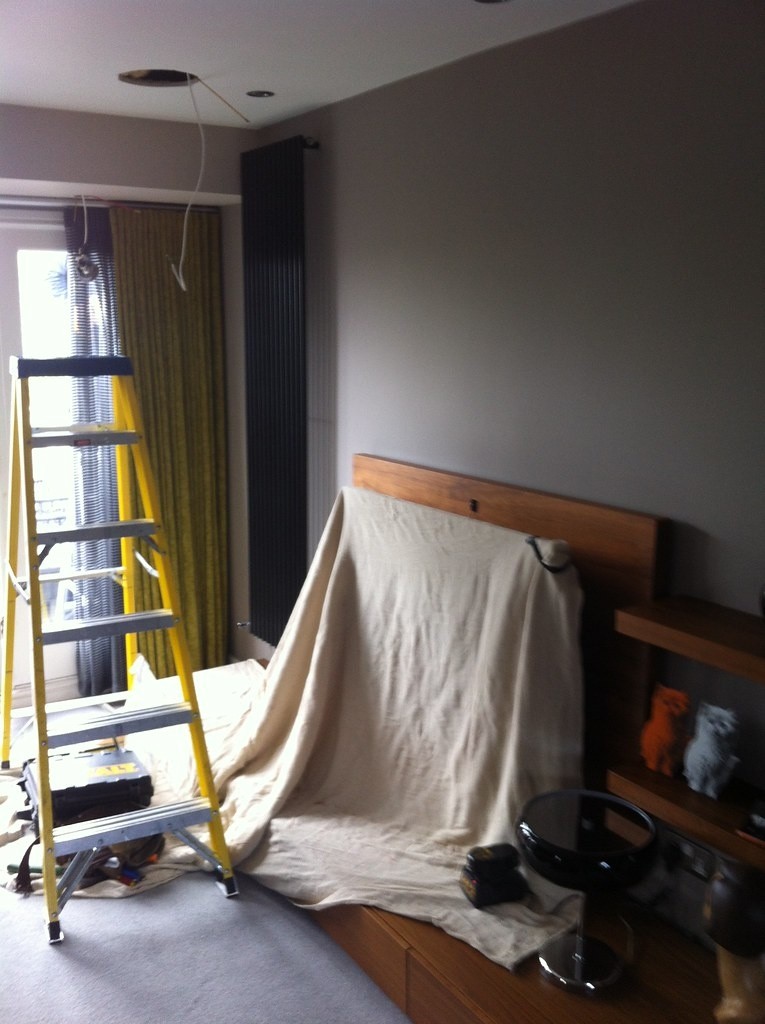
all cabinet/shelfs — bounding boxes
[608,594,764,872]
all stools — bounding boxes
[515,791,657,1000]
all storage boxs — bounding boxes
[19,747,155,837]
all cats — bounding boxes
[682,701,739,801]
[638,682,691,781]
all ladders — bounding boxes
[3,355,239,945]
